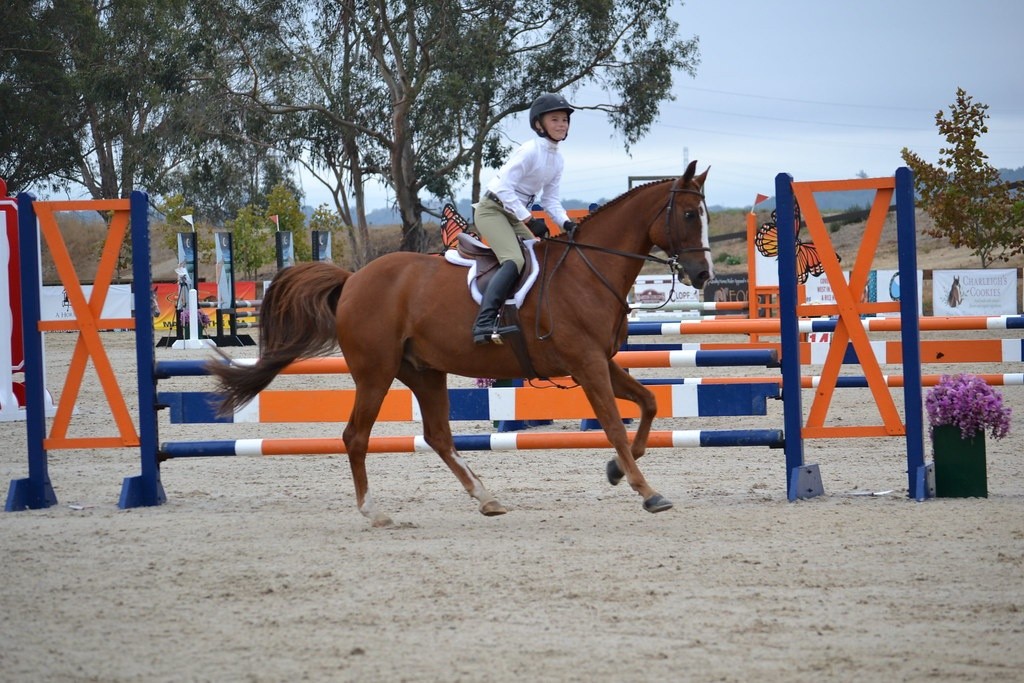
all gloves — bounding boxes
[525,217,548,237]
[564,220,578,230]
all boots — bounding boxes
[472,261,520,345]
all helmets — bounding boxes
[529,94,574,137]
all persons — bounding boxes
[472,95,580,343]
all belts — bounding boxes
[484,190,505,206]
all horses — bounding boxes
[195,158,717,531]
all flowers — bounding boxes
[179,309,212,328]
[923,372,1015,440]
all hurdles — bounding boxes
[8,191,937,519]
[171,280,272,349]
